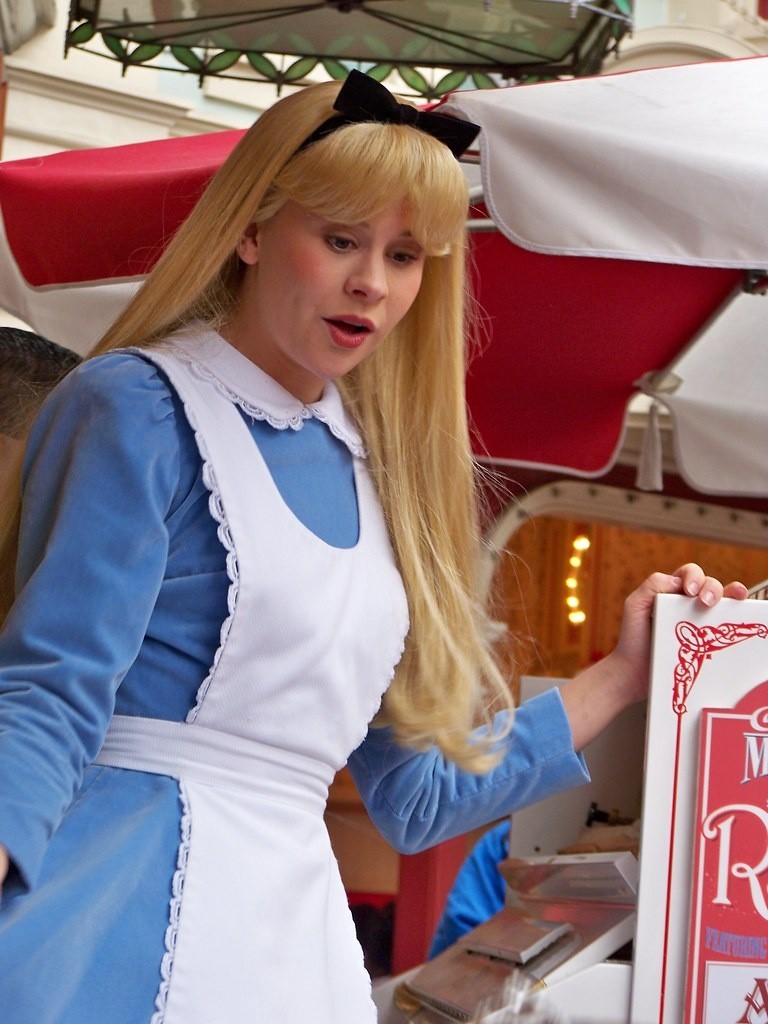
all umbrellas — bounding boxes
[1,0,767,502]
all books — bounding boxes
[410,907,581,1021]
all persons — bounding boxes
[1,72,747,1024]
[429,819,511,961]
